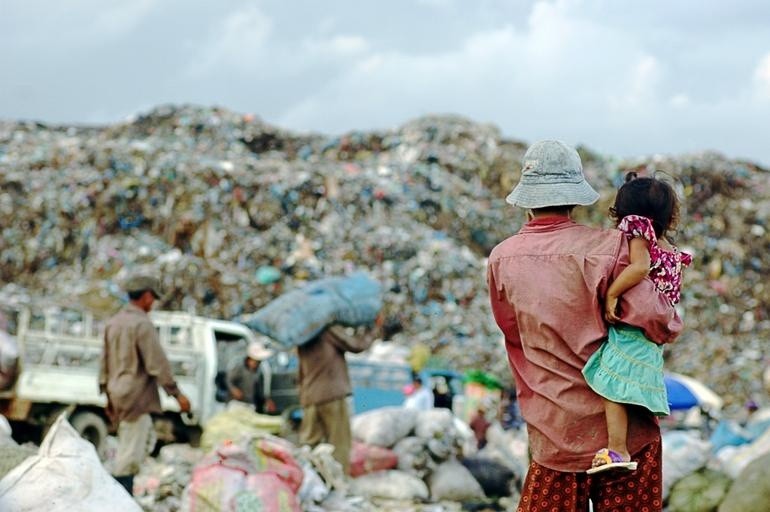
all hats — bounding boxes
[127,277,163,300]
[505,139,600,209]
[246,342,275,361]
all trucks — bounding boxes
[14,309,274,458]
[277,360,468,431]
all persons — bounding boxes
[470,406,491,449]
[224,343,274,416]
[401,377,435,411]
[298,313,384,478]
[586,169,691,474]
[96,275,190,498]
[487,139,684,512]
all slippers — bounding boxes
[585,448,639,475]
